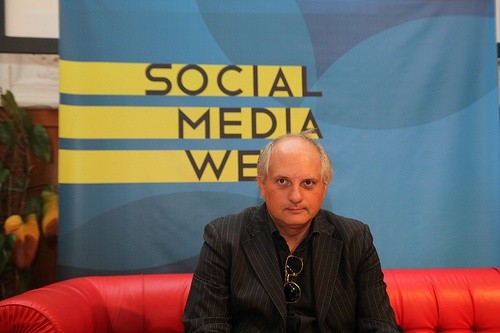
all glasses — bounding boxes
[283,255,303,304]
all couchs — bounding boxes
[0,268,500,333]
